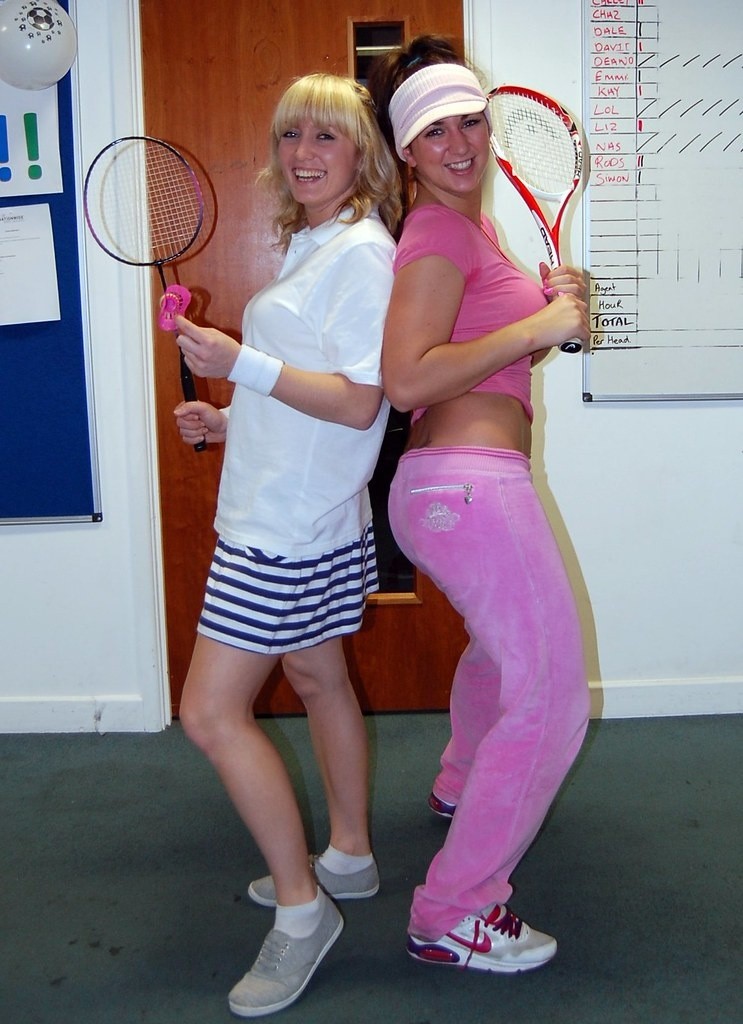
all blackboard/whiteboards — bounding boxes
[579,0,743,401]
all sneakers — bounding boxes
[405,902,558,973]
[247,852,380,908]
[425,787,456,818]
[226,893,345,1019]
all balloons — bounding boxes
[0,0,79,91]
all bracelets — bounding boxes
[225,344,283,399]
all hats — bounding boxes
[388,63,489,162]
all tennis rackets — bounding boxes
[486,85,583,353]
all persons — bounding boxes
[174,75,397,1017]
[371,34,592,973]
